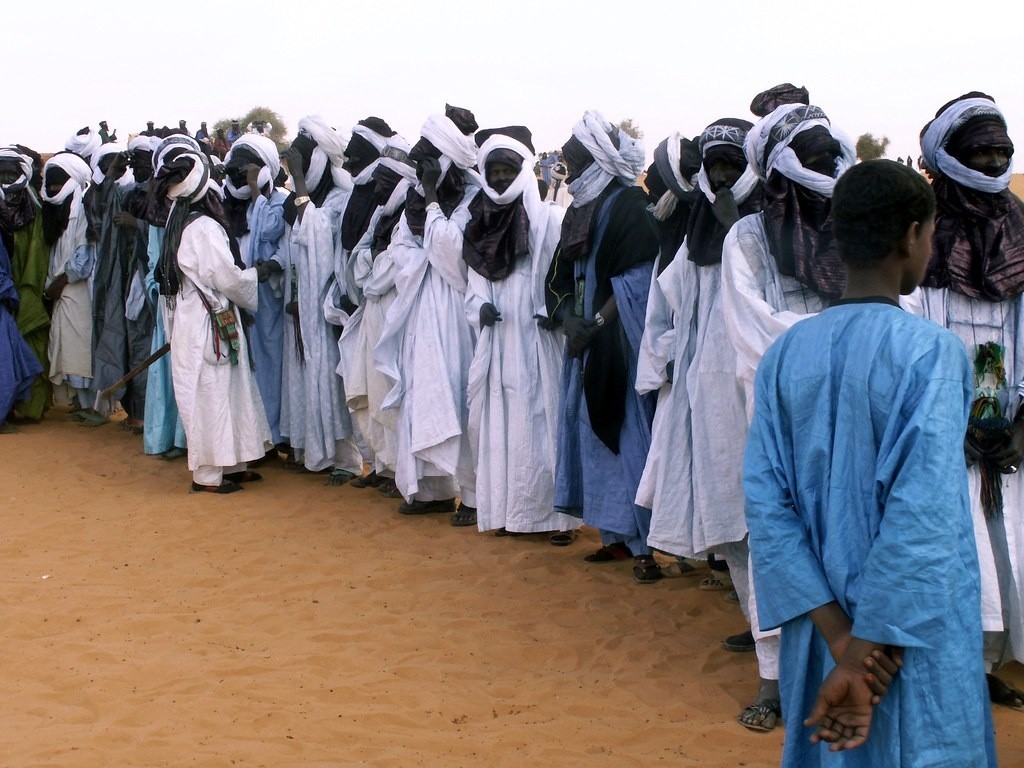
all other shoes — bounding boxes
[192,480,242,494]
[223,471,262,483]
[352,470,381,489]
[158,447,186,460]
[378,478,402,498]
[986,673,1024,710]
[122,419,144,434]
[699,570,733,590]
[724,631,755,651]
[725,590,739,603]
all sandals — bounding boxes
[497,526,519,537]
[549,529,576,545]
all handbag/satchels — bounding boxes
[212,310,238,345]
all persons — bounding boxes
[739,158,999,768]
[0,82,1024,731]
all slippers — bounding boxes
[739,704,780,731]
[451,502,477,527]
[67,410,94,422]
[398,498,456,514]
[82,416,110,427]
[586,543,631,563]
[325,470,353,486]
[632,559,661,584]
